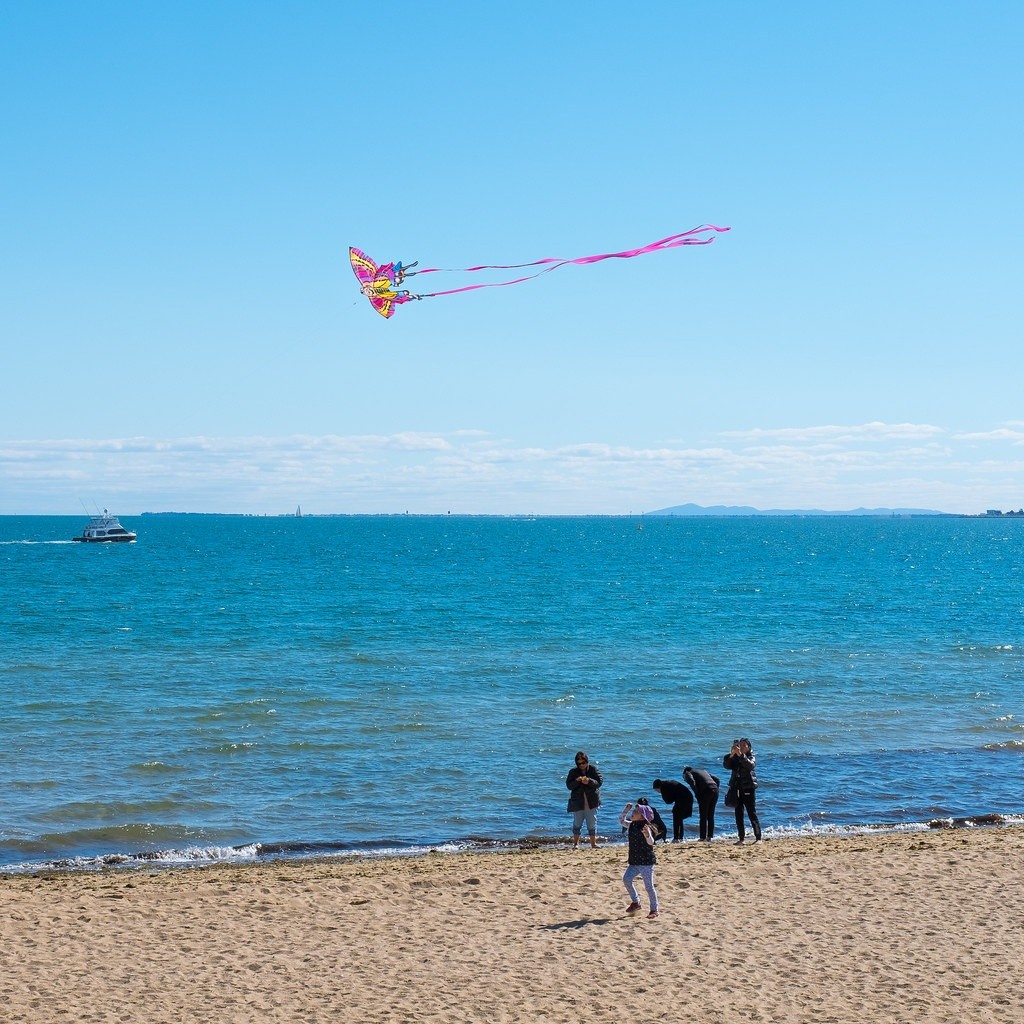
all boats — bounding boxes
[72,496,136,542]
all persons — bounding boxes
[619,803,659,918]
[622,798,667,845]
[566,751,603,850]
[723,738,762,845]
[682,767,720,842]
[653,779,693,843]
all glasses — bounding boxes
[577,760,586,766]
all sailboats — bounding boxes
[294,505,303,518]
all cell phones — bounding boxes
[734,739,740,748]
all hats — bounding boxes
[635,803,654,825]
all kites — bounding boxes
[349,223,732,320]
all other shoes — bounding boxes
[733,839,746,845]
[645,911,660,920]
[749,839,763,847]
[670,839,684,844]
[626,901,642,913]
[697,837,711,842]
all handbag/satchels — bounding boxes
[725,788,735,807]
